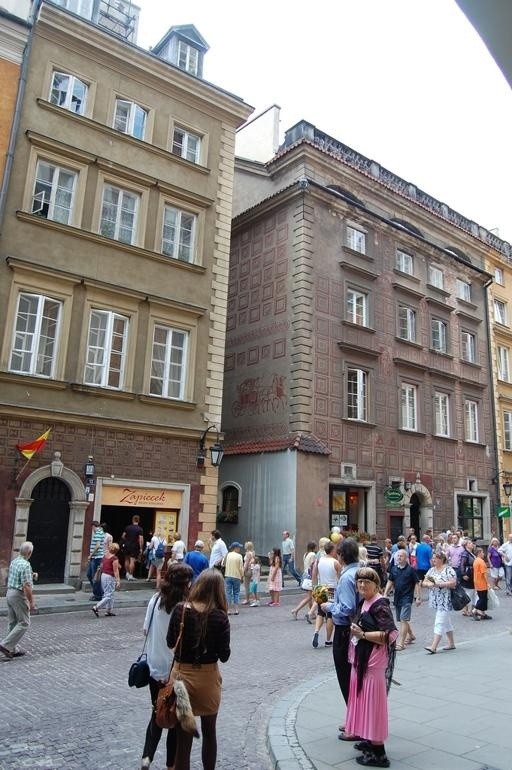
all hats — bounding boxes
[231,542,243,547]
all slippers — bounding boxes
[425,647,436,653]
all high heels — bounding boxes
[292,610,316,624]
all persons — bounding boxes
[382,548,422,651]
[291,525,512,623]
[0,541,38,658]
[87,515,301,617]
[319,539,367,742]
[344,566,399,767]
[311,543,341,649]
[139,563,196,769]
[421,550,456,653]
[161,566,231,770]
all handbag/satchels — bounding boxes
[487,588,500,609]
[451,584,471,610]
[155,686,177,728]
[128,654,150,688]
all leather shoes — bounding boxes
[339,727,390,767]
[0,645,25,658]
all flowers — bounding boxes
[311,583,329,620]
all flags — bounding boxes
[14,427,52,459]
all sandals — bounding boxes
[92,609,99,617]
[105,612,115,616]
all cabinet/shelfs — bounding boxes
[150,548,172,578]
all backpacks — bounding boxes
[155,539,165,557]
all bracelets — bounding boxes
[362,630,366,640]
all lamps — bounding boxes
[85,460,95,478]
[382,480,412,492]
[492,470,512,498]
[198,425,224,467]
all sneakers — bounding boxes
[242,599,280,607]
[313,633,318,647]
[325,642,333,647]
